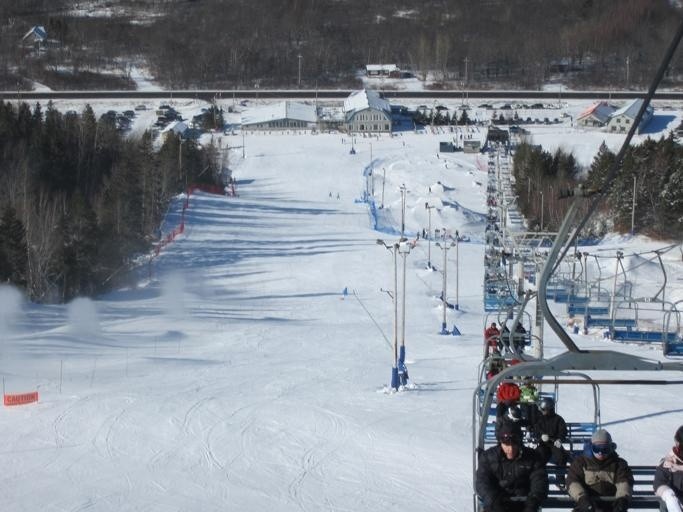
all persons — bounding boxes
[483,321,569,491]
[475,421,547,512]
[564,429,634,512]
[653,426,683,511]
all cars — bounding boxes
[116,104,176,131]
[416,103,544,110]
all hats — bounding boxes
[591,428,612,446]
[540,398,554,411]
[498,422,522,439]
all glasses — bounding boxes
[593,447,611,454]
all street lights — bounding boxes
[297,54,304,86]
[425,201,435,271]
[435,227,460,335]
[448,230,471,310]
[376,231,420,389]
[398,183,407,241]
